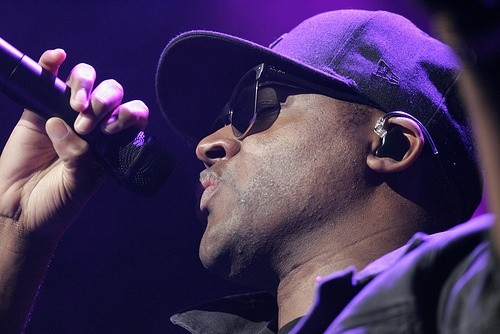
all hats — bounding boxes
[155,9,484,224]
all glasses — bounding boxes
[208,63,388,141]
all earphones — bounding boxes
[375,126,410,161]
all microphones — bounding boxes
[0,37,164,195]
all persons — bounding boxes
[0,0,500,334]
